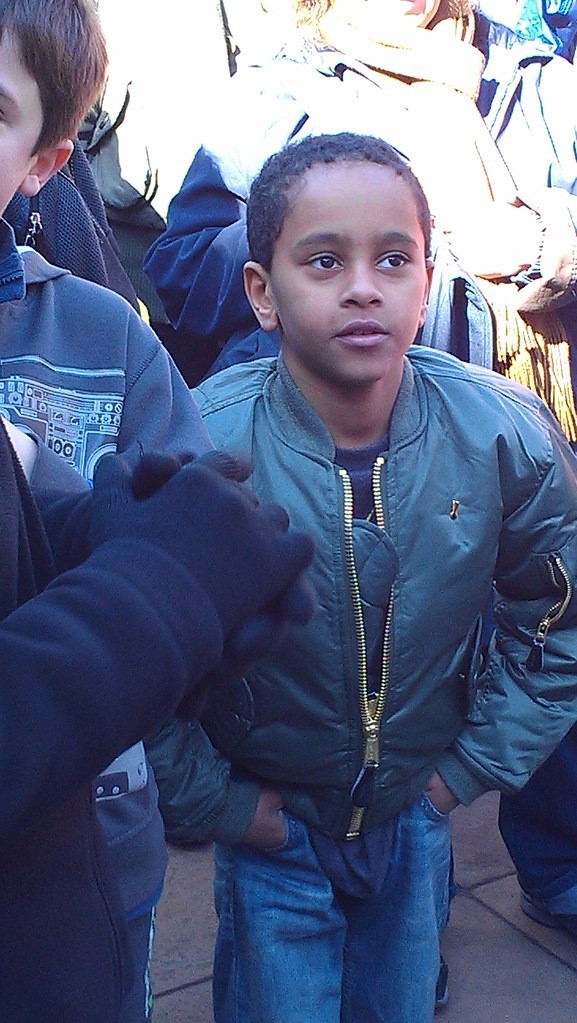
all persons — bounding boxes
[0,0,577,1023]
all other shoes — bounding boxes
[434,953,449,1010]
[521,889,577,935]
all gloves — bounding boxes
[87,449,316,680]
